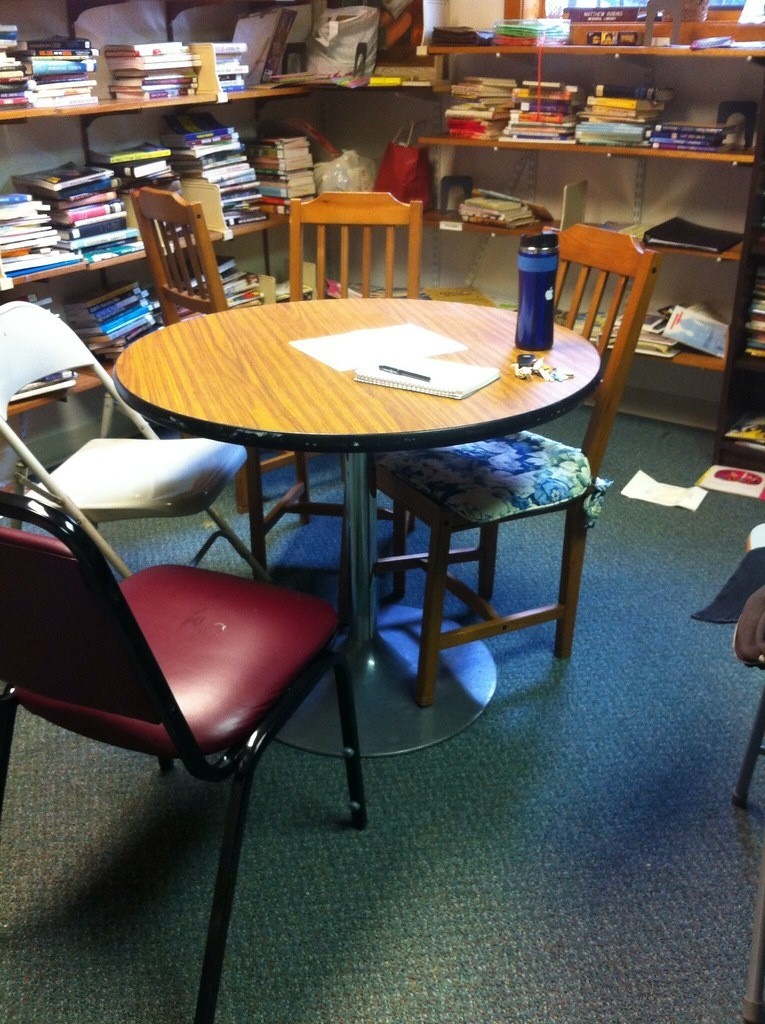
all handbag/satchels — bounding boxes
[373,119,434,212]
[302,6,380,89]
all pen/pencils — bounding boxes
[378,365,430,383]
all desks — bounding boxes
[112,296,606,756]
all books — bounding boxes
[323,278,733,359]
[0,24,252,112]
[458,188,555,227]
[444,76,731,155]
[0,112,318,291]
[6,253,319,404]
[354,356,501,399]
[725,402,765,452]
[745,265,765,357]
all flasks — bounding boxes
[515,233,560,351]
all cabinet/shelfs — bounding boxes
[713,130,764,477]
[417,43,764,432]
[0,81,318,418]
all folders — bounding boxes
[642,215,745,255]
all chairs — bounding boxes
[128,186,346,515]
[0,491,371,1024]
[241,191,427,597]
[0,298,274,585]
[372,223,660,709]
